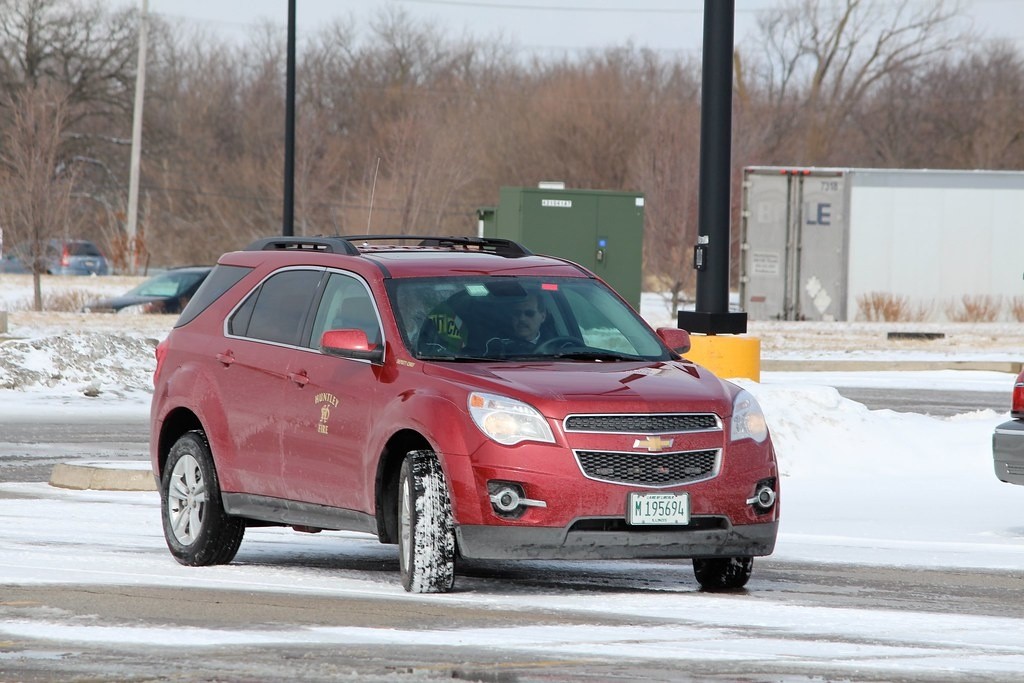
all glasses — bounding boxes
[511,309,539,317]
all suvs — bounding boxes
[149,231,781,595]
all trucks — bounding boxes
[738,164,1024,325]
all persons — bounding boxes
[395,279,465,357]
[488,292,565,357]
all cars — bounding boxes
[81,264,216,314]
[0,237,109,277]
